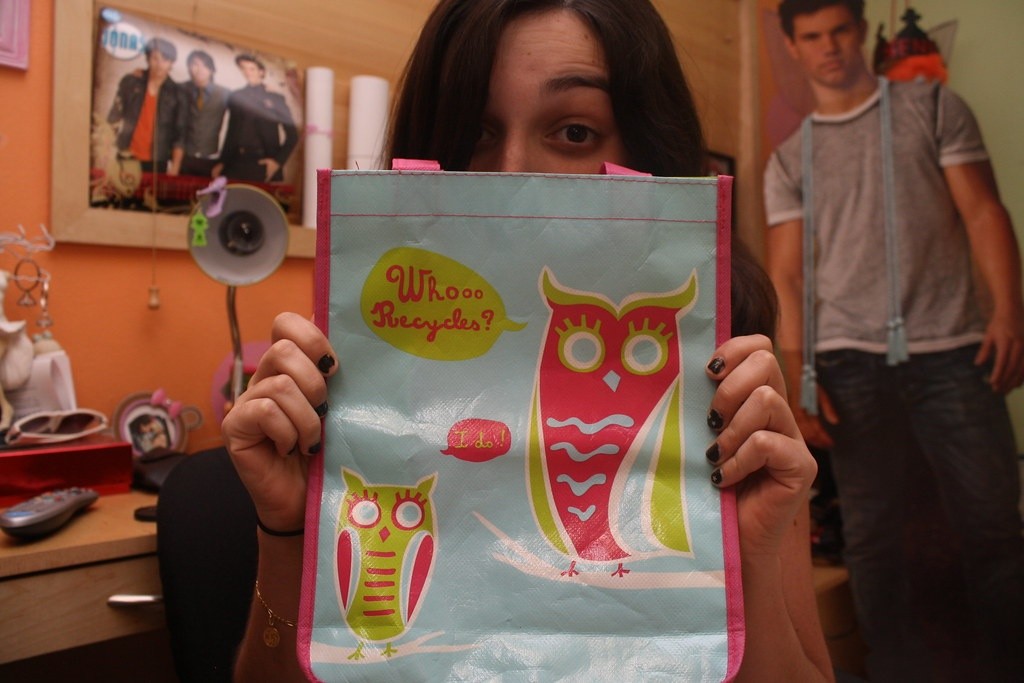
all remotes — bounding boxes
[1,484,96,544]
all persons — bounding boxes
[765,0,1024,683]
[223,0,832,683]
[134,414,167,450]
[108,37,300,214]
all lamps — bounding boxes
[187,185,290,414]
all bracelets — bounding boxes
[256,580,297,647]
[254,513,304,536]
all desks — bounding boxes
[0,486,177,683]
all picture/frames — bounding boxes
[51,0,401,258]
